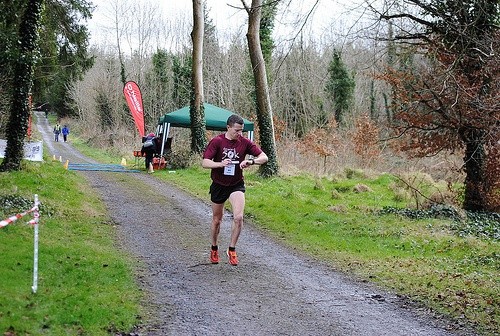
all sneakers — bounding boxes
[226,250,238,265]
[209,249,218,263]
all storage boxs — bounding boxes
[152,157,165,170]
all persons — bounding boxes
[62,125,69,142]
[141,133,161,172]
[202,115,268,265]
[53,122,61,142]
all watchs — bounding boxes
[249,158,255,165]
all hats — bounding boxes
[148,133,154,136]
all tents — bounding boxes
[157,103,254,165]
